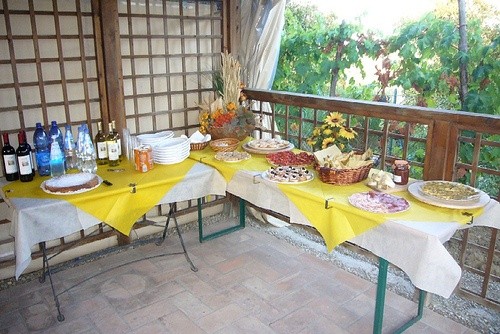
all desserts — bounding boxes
[250,139,290,148]
[267,164,312,183]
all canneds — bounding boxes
[133,145,154,172]
[392,160,411,185]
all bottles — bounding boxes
[112,121,122,157]
[105,123,120,167]
[2,132,19,181]
[33,120,98,177]
[19,130,35,177]
[95,121,108,165]
[16,133,33,182]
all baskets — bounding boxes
[209,138,239,153]
[190,143,207,151]
[316,164,372,184]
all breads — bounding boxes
[323,152,356,170]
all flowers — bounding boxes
[305,112,358,152]
[193,50,257,139]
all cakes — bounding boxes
[44,173,98,193]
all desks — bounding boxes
[0,152,227,323]
[187,137,495,334]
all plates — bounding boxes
[136,131,191,165]
[347,192,410,214]
[40,174,103,195]
[214,151,252,163]
[265,157,313,169]
[408,180,490,209]
[261,169,314,184]
[241,140,294,155]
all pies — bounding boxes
[422,182,477,199]
